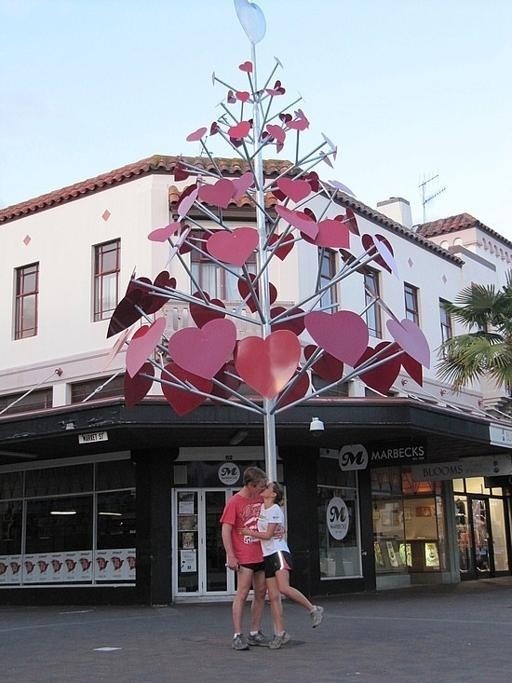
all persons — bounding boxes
[236,480,325,649]
[218,464,272,650]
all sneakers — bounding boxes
[311,606,324,628]
[232,630,291,649]
